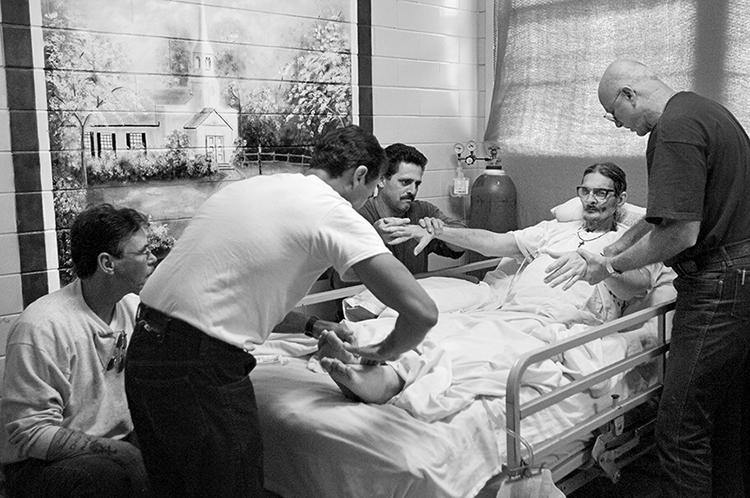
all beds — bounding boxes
[210,250,679,498]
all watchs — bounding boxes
[304,315,318,336]
[605,257,623,280]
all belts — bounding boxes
[671,241,750,276]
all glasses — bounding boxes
[603,91,627,125]
[107,242,154,261]
[576,184,624,202]
[106,329,128,373]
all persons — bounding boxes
[583,58,750,497]
[318,161,652,405]
[353,144,467,273]
[0,203,157,498]
[123,125,438,498]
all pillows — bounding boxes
[548,195,649,236]
